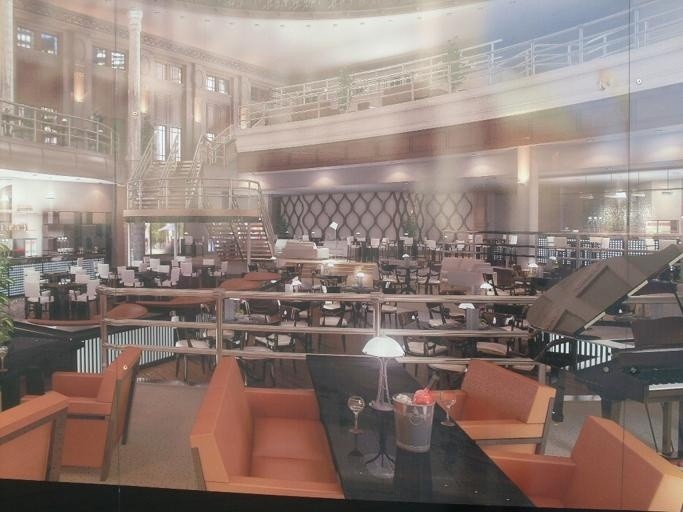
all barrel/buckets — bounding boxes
[392,393,436,454]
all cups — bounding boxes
[436,390,456,427]
[348,396,365,434]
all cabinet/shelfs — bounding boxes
[42,210,112,255]
[0,253,106,298]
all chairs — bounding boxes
[149,259,161,267]
[158,265,170,273]
[317,311,348,352]
[97,263,110,280]
[117,265,126,279]
[162,268,180,287]
[214,262,228,279]
[422,341,467,389]
[202,258,214,265]
[180,262,193,276]
[231,330,277,389]
[397,310,451,384]
[174,327,214,383]
[254,332,298,373]
[415,270,441,294]
[138,263,148,273]
[121,270,135,287]
[426,302,457,328]
[23,276,47,320]
[72,279,100,320]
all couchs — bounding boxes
[190,356,345,500]
[482,414,683,512]
[430,357,557,455]
[19,346,142,482]
[0,390,70,482]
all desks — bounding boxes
[43,281,87,319]
[362,304,398,328]
[192,264,215,288]
[134,271,165,288]
[304,353,535,508]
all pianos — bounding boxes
[527,243,683,457]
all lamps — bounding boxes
[457,303,475,321]
[527,262,538,268]
[363,414,397,479]
[479,282,493,295]
[362,332,406,413]
[356,272,364,285]
[402,253,410,258]
